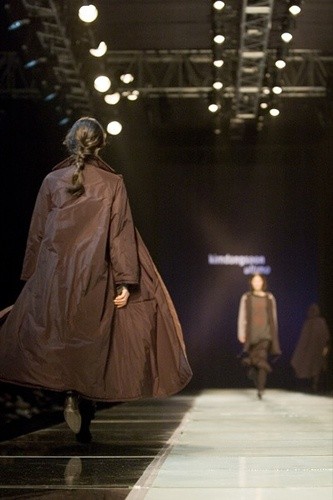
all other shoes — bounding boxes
[64,390,84,434]
[76,425,92,443]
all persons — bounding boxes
[0,116,192,445]
[290,304,330,392]
[238,272,282,402]
